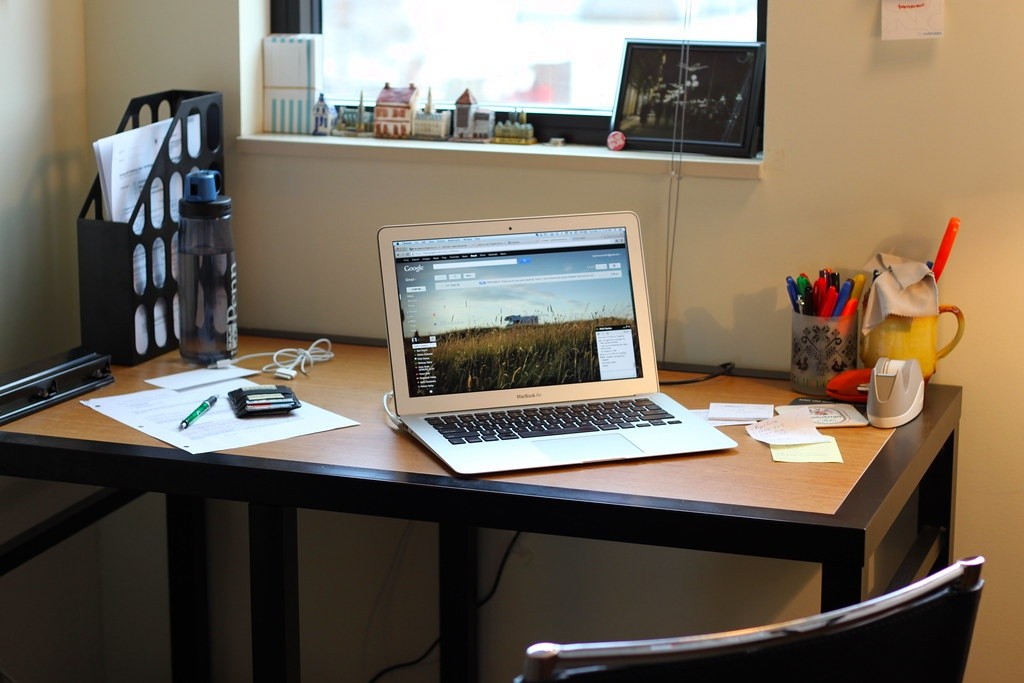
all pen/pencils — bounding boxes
[178,393,221,432]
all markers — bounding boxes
[784,268,867,337]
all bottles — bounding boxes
[177,169,237,366]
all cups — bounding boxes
[859,287,965,386]
[790,310,859,396]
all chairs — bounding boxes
[511,555,986,683]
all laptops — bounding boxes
[377,211,738,477]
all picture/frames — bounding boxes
[609,37,766,159]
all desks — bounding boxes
[0,326,964,683]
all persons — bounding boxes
[636,98,664,128]
